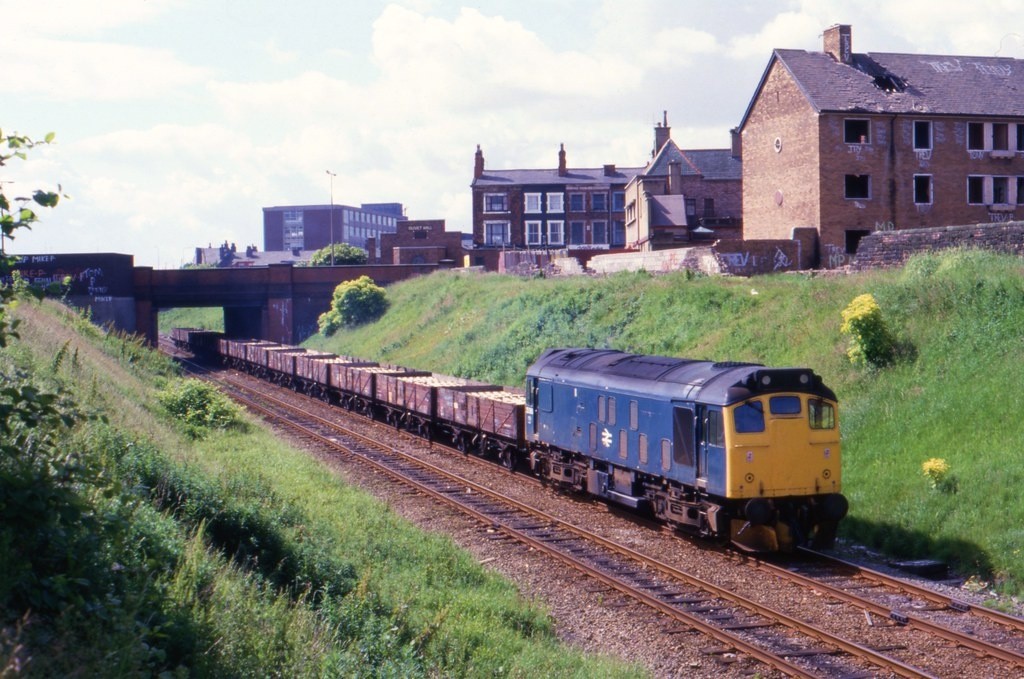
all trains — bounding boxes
[170,326,848,557]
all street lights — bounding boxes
[326,170,336,267]
